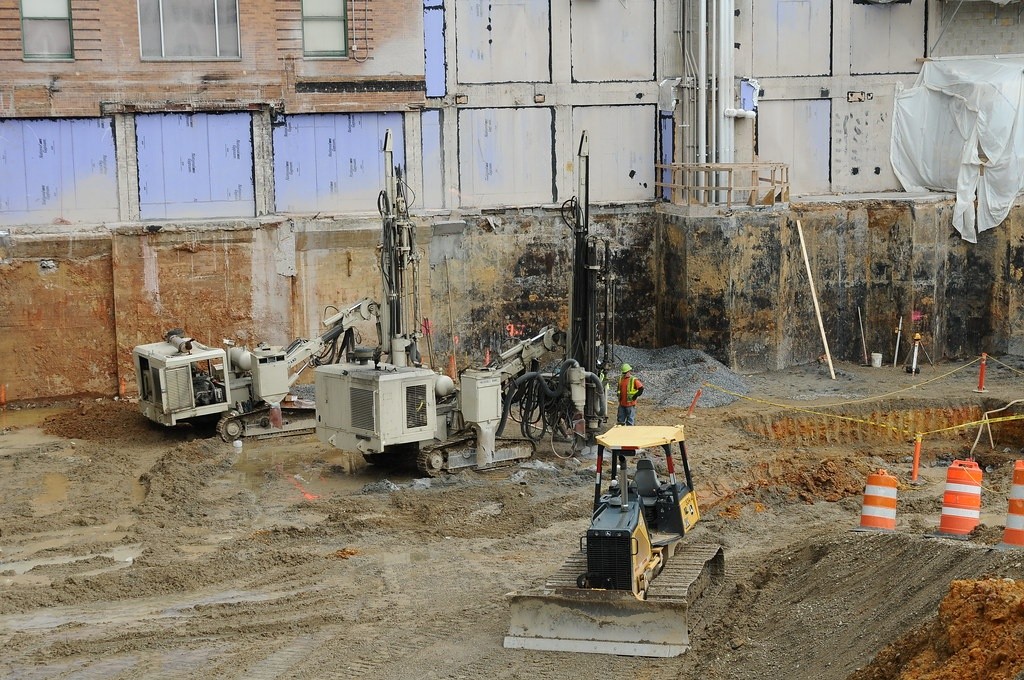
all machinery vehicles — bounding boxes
[314,127,612,479]
[504,424,724,658]
[132,130,420,445]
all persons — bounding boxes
[616,363,644,426]
[408,395,425,427]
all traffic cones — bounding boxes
[446,354,457,383]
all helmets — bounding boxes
[621,363,633,374]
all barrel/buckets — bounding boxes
[870,353,882,368]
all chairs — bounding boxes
[632,459,661,506]
